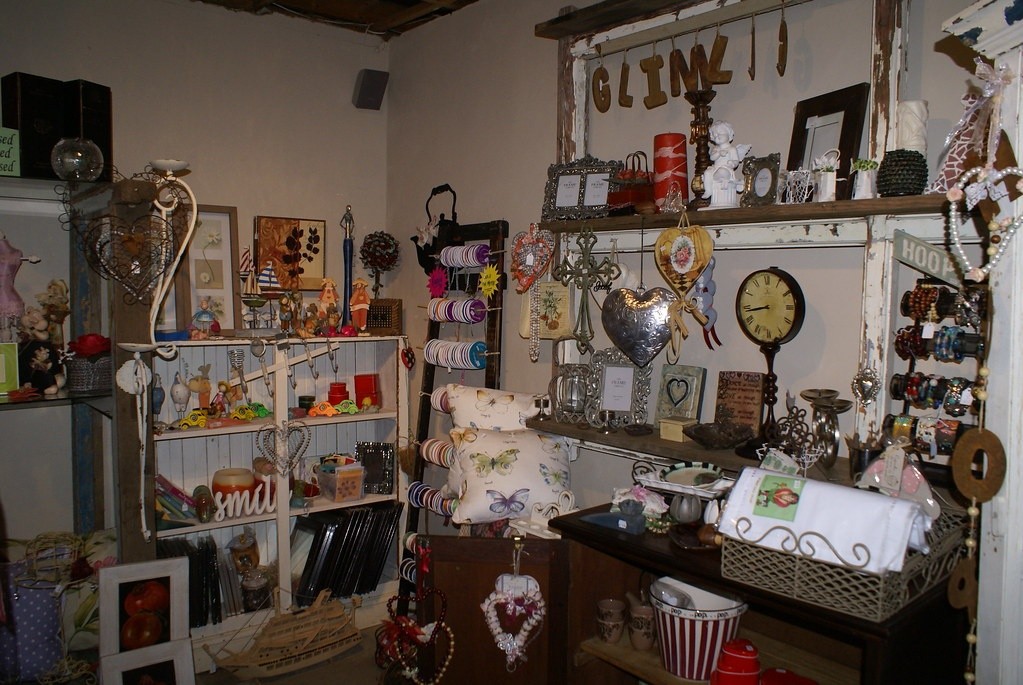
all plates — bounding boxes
[634,469,736,502]
[659,461,724,488]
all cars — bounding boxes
[180,410,207,431]
[230,402,269,421]
[309,400,358,417]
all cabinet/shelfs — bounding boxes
[392,211,509,629]
[413,533,970,685]
[152,335,410,672]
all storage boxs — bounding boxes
[658,416,700,442]
[317,467,368,504]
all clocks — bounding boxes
[734,266,806,461]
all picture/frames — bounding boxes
[172,203,242,337]
[584,346,652,434]
[541,153,619,223]
[355,440,395,494]
[98,555,191,656]
[99,636,195,685]
[740,152,780,208]
[780,81,870,204]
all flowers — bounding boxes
[359,230,400,298]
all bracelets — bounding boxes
[893,325,931,361]
[905,371,946,410]
[953,282,982,327]
[934,419,963,454]
[934,325,966,365]
[917,417,938,446]
[909,284,950,324]
[892,413,917,444]
[943,376,971,416]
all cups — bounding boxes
[241,579,271,613]
[595,598,657,652]
[230,536,259,574]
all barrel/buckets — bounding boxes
[649,579,750,682]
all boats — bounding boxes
[202,586,363,681]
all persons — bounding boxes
[759,487,799,508]
[291,291,302,331]
[43,279,70,348]
[702,119,740,200]
[317,277,339,314]
[215,381,231,405]
[277,294,293,334]
[350,278,370,331]
[191,298,219,336]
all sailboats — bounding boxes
[237,245,285,307]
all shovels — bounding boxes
[317,318,334,360]
[275,333,294,377]
